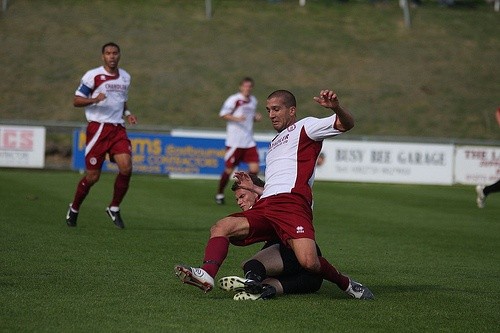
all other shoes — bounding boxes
[215,194,225,206]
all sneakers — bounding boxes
[105,206,125,229]
[217,275,263,295]
[66,202,79,226]
[174,263,215,293]
[341,273,375,301]
[475,185,487,209]
[232,284,273,301]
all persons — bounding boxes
[218,175,323,301]
[476,107,500,210]
[65,43,138,228]
[215,77,263,206]
[174,90,374,301]
